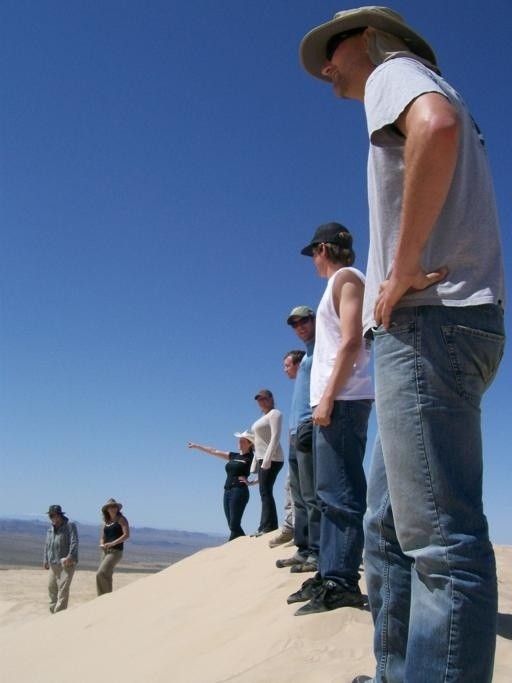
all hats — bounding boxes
[234,431,255,446]
[47,505,65,514]
[102,498,122,512]
[255,390,272,400]
[301,223,350,256]
[301,6,435,83]
[287,306,314,324]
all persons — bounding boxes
[276,304,319,571]
[94,498,130,597]
[186,428,256,541]
[43,505,79,615]
[285,221,375,618]
[249,388,283,536]
[268,348,304,547]
[300,5,505,683]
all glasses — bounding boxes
[291,317,309,328]
[327,26,367,61]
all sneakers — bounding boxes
[277,553,364,616]
[270,533,295,547]
